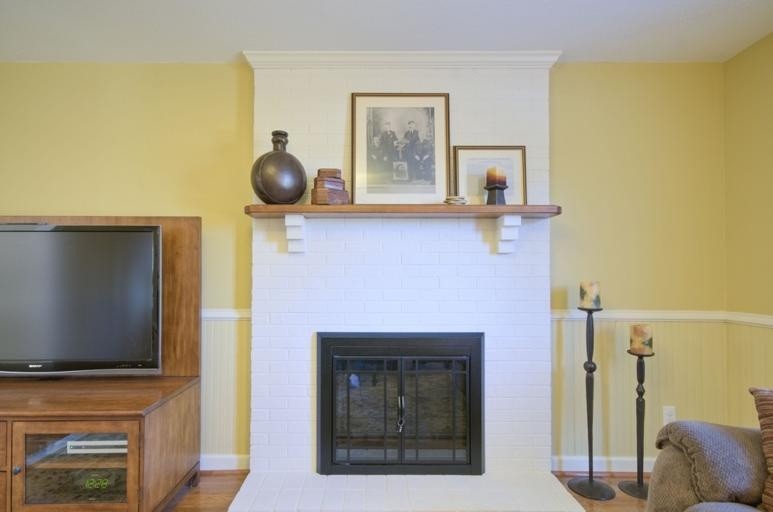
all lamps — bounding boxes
[643,418,765,512]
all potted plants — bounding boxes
[348,90,451,206]
[451,143,527,207]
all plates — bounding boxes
[0,374,203,511]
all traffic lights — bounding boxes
[747,385,772,512]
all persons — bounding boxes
[370,121,432,181]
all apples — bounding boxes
[577,279,602,311]
[485,164,508,187]
[628,323,654,356]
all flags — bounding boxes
[248,129,307,205]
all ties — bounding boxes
[0,223,162,380]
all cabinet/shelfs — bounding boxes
[251,130,308,207]
